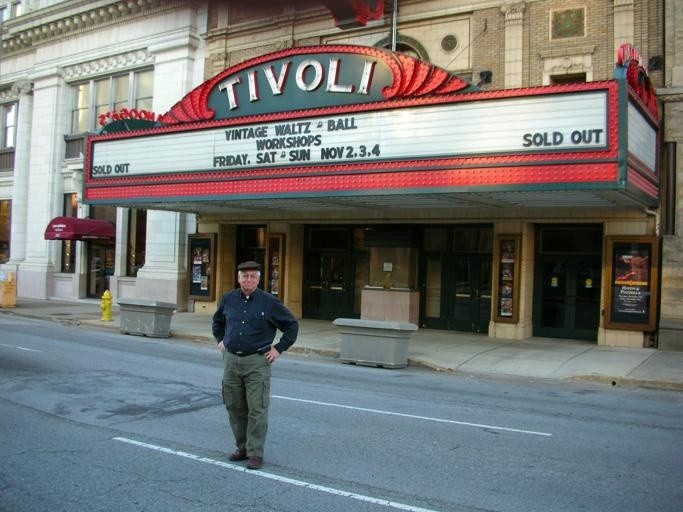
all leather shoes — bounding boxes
[246,456,262,469]
[229,449,246,461]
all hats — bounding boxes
[237,260,261,272]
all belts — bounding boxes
[227,348,257,357]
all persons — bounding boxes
[211,261,298,471]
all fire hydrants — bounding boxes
[99,289,114,322]
